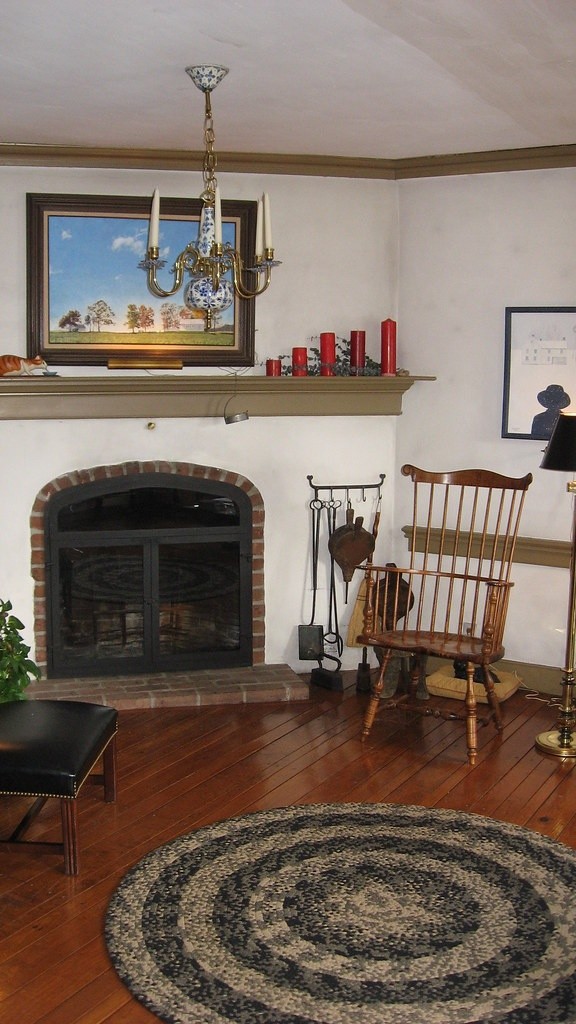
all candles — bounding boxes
[381,318,396,377]
[292,347,308,376]
[266,360,282,376]
[350,330,365,375]
[320,333,336,377]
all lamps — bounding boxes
[143,66,272,336]
[538,413,575,760]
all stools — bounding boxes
[0,701,118,877]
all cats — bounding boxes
[0,355,48,376]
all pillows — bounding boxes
[425,664,524,703]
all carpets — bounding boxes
[105,801,576,1024]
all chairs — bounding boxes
[352,465,533,766]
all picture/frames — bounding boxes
[25,193,258,367]
[501,306,575,440]
